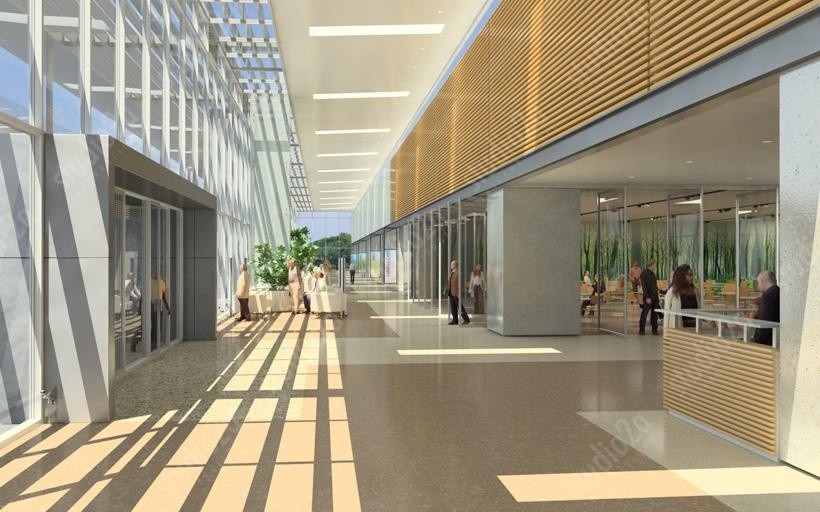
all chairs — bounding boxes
[253,267,349,319]
[581,271,763,328]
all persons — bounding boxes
[581,269,604,317]
[126,273,141,316]
[663,264,700,334]
[630,263,642,290]
[442,260,470,325]
[469,262,486,314]
[235,264,251,322]
[288,259,301,313]
[749,270,780,345]
[350,263,355,284]
[639,258,661,336]
[303,272,324,313]
[131,266,172,351]
